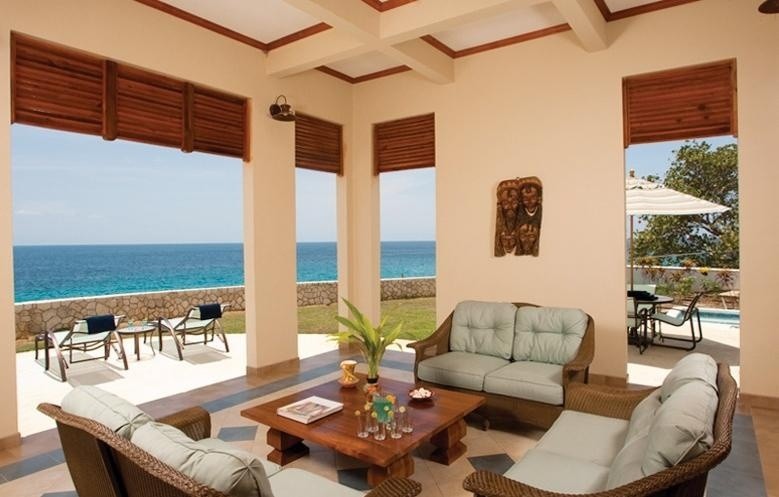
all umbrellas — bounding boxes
[625,169,732,291]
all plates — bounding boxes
[408,389,435,401]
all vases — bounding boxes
[336,359,361,389]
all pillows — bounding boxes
[622,351,719,444]
[603,380,720,492]
[130,419,274,497]
[449,299,519,361]
[61,384,154,443]
[512,306,590,365]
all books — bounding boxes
[277,394,343,425]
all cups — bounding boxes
[354,392,414,442]
[127,318,150,332]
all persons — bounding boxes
[494,176,541,255]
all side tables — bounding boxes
[116,324,156,361]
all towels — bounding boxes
[191,304,222,321]
[81,314,116,334]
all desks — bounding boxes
[717,291,740,308]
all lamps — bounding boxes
[269,92,297,121]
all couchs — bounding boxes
[406,302,596,431]
[462,360,738,497]
[35,401,424,497]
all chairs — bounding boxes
[625,282,704,355]
[143,301,232,361]
[34,315,128,382]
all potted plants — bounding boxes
[325,295,411,404]
